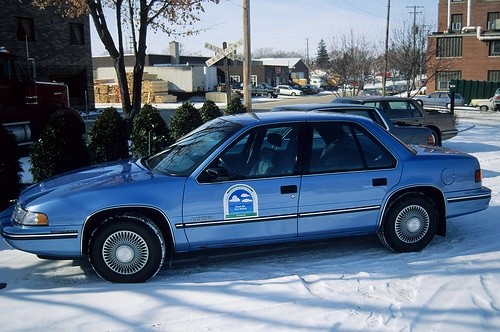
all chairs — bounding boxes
[248,134,283,176]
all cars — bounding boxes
[274,85,303,96]
[412,91,465,109]
[469,88,500,111]
[0,110,491,283]
[330,97,458,147]
[223,104,434,153]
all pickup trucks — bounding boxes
[251,82,279,98]
